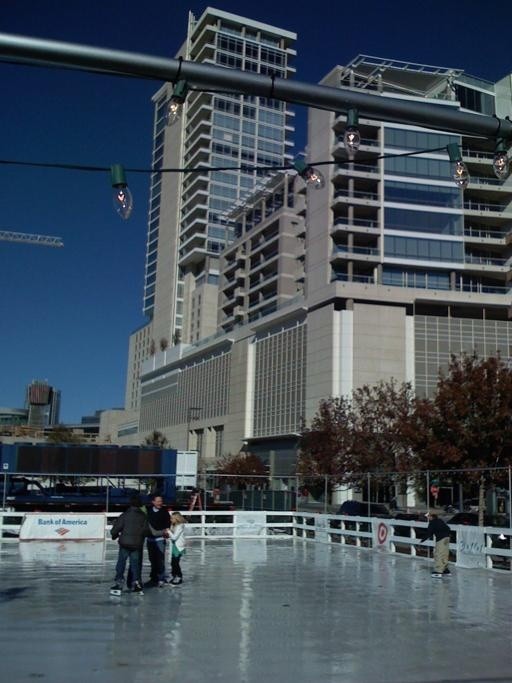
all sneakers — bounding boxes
[109,577,182,591]
[431,569,450,574]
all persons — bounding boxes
[165,510,187,585]
[420,512,451,575]
[109,493,152,593]
[124,496,170,589]
[142,492,172,588]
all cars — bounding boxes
[328,499,512,576]
